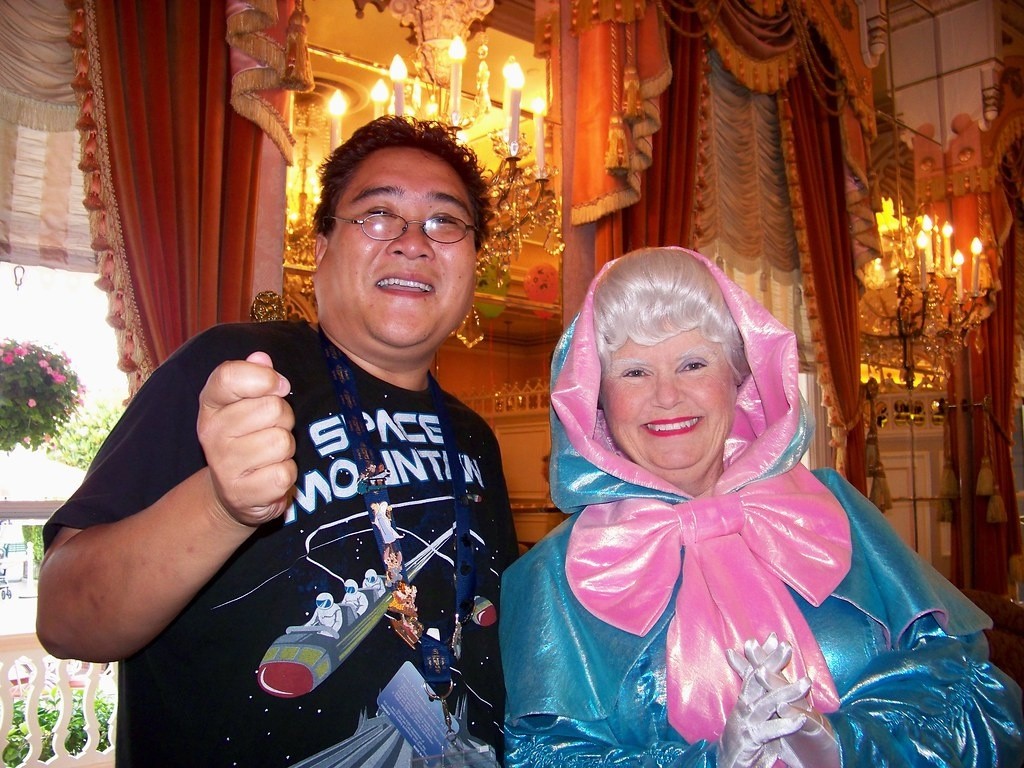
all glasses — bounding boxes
[325,211,480,244]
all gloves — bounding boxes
[714,632,841,768]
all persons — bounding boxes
[35,115,518,768]
[500,245,1024,768]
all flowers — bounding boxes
[0,338,86,451]
[0,396,0,397]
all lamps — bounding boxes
[849,123,995,415]
[284,0,562,344]
